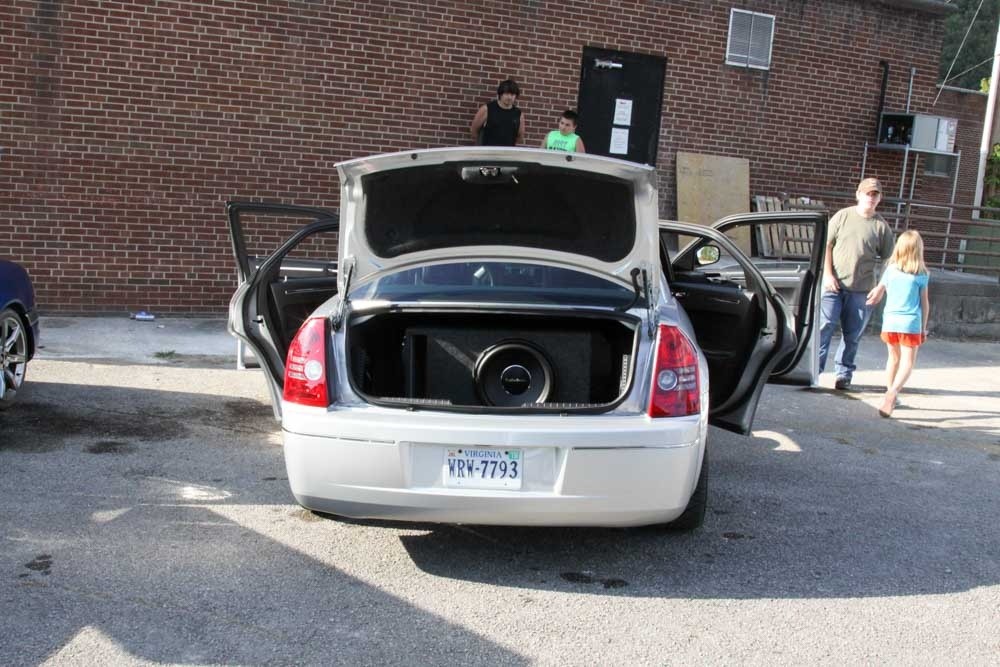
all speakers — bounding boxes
[403,329,596,407]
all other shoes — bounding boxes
[879,388,896,418]
[896,399,900,406]
[835,373,851,390]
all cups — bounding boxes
[136,314,154,321]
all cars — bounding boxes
[0,257,39,412]
[224,143,832,537]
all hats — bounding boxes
[858,177,882,195]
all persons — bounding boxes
[879,230,931,418]
[471,80,525,147]
[540,110,585,153]
[821,178,897,390]
